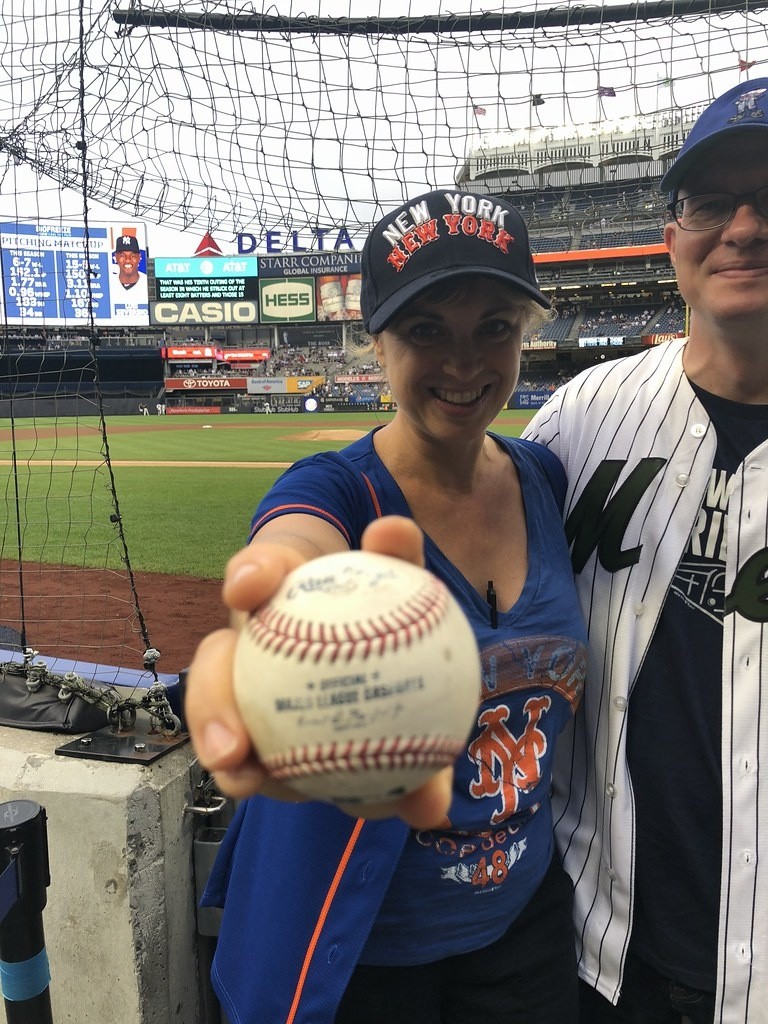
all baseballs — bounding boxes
[227,551,486,807]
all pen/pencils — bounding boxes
[487,580,498,629]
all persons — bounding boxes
[519,77,768,1024]
[183,188,593,1024]
[15,328,381,415]
[110,235,148,305]
[507,187,691,392]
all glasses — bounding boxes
[667,185,768,231]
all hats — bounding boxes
[659,77,768,192]
[112,236,141,254]
[359,189,551,334]
